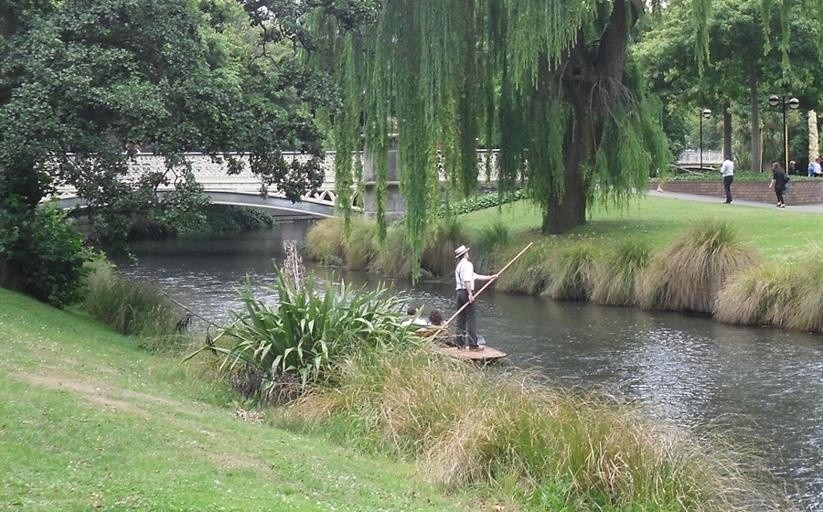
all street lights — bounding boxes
[769,94,800,174]
[699,108,712,167]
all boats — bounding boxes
[406,329,507,366]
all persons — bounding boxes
[427,308,448,331]
[399,306,427,328]
[788,155,823,178]
[656,167,665,193]
[453,245,501,353]
[719,154,734,205]
[768,162,789,208]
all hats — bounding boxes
[454,244,470,259]
